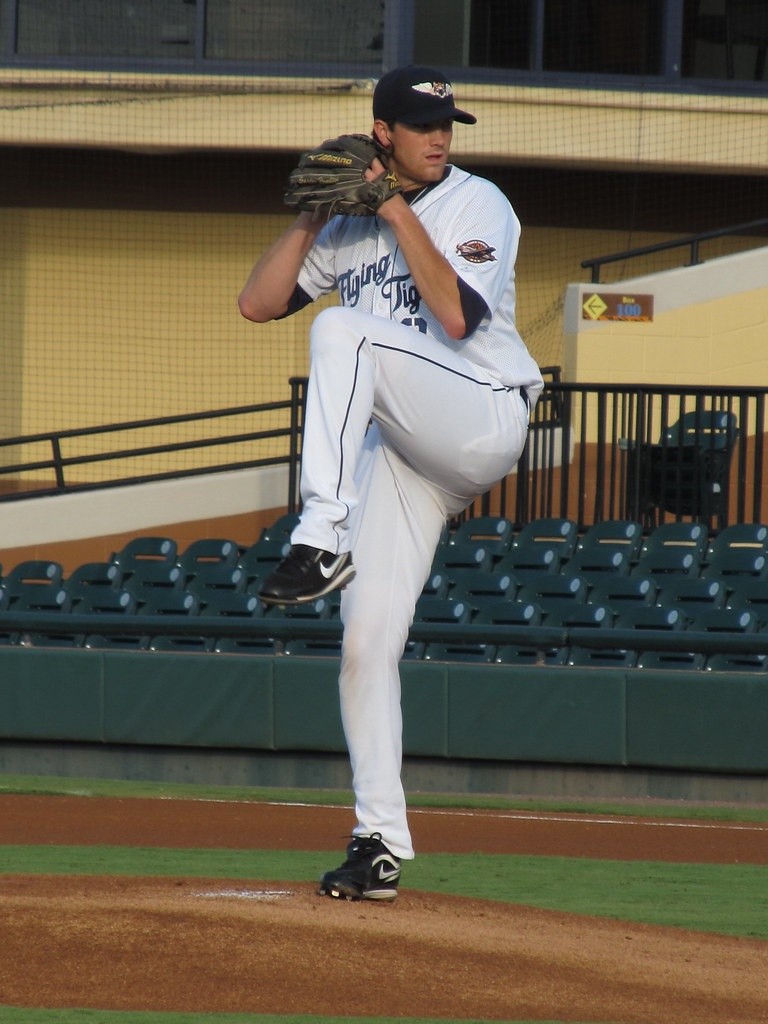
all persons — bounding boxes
[238,66,546,903]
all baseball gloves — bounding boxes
[283,132,404,224]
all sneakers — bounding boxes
[258,543,356,605]
[317,837,402,901]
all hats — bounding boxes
[373,64,478,126]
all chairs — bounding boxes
[618,409,738,529]
[1,509,767,674]
